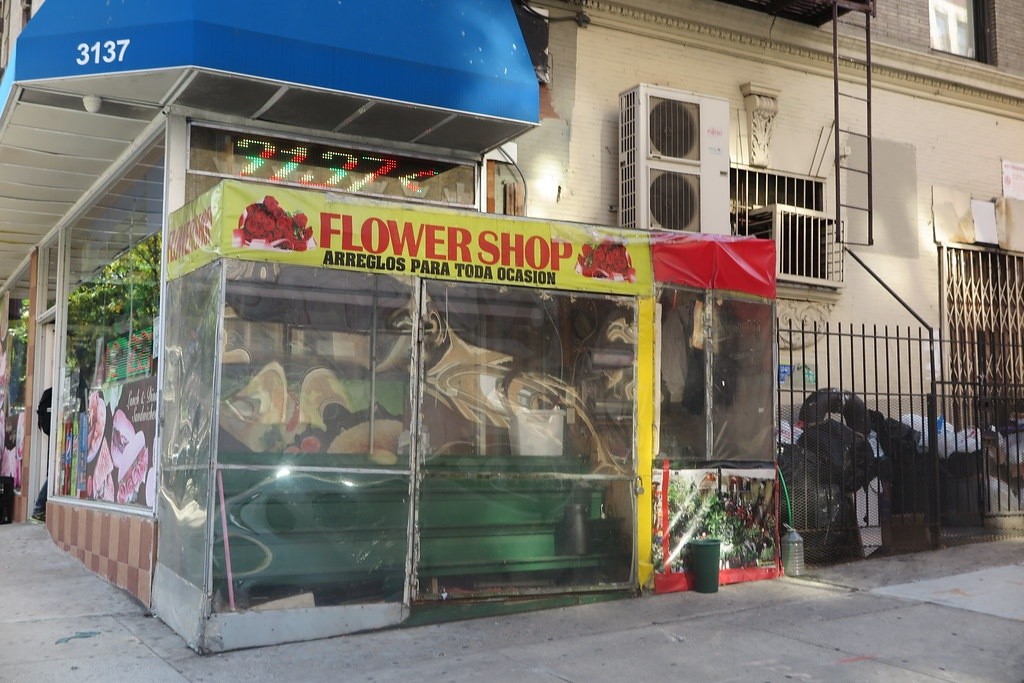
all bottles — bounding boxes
[781,522,805,577]
[562,502,589,557]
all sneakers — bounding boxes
[29,511,45,524]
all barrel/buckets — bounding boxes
[688,538,723,594]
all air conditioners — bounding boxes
[739,205,848,288]
[618,82,733,235]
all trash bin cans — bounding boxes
[688,539,723,593]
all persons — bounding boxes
[29,387,52,525]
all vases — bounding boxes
[685,538,721,592]
